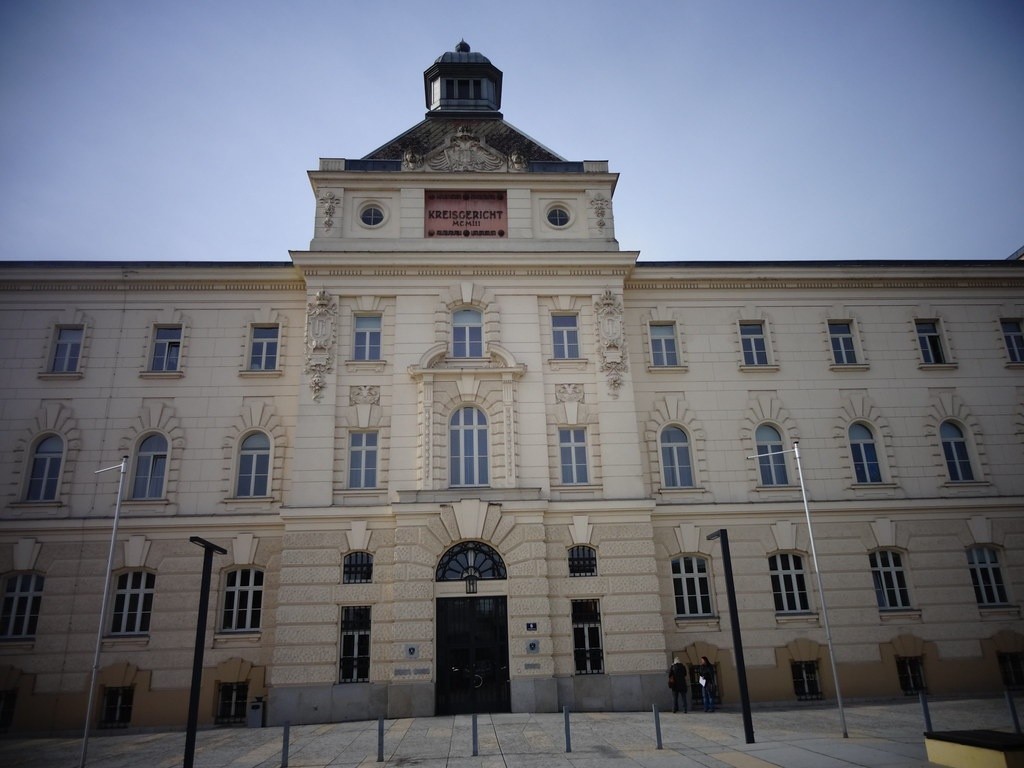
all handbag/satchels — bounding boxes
[668,675,675,689]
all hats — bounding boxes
[674,657,680,664]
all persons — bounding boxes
[668,657,687,713]
[698,656,715,712]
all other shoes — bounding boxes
[705,708,715,712]
[684,711,687,713]
[672,711,676,713]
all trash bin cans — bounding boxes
[246,701,264,727]
